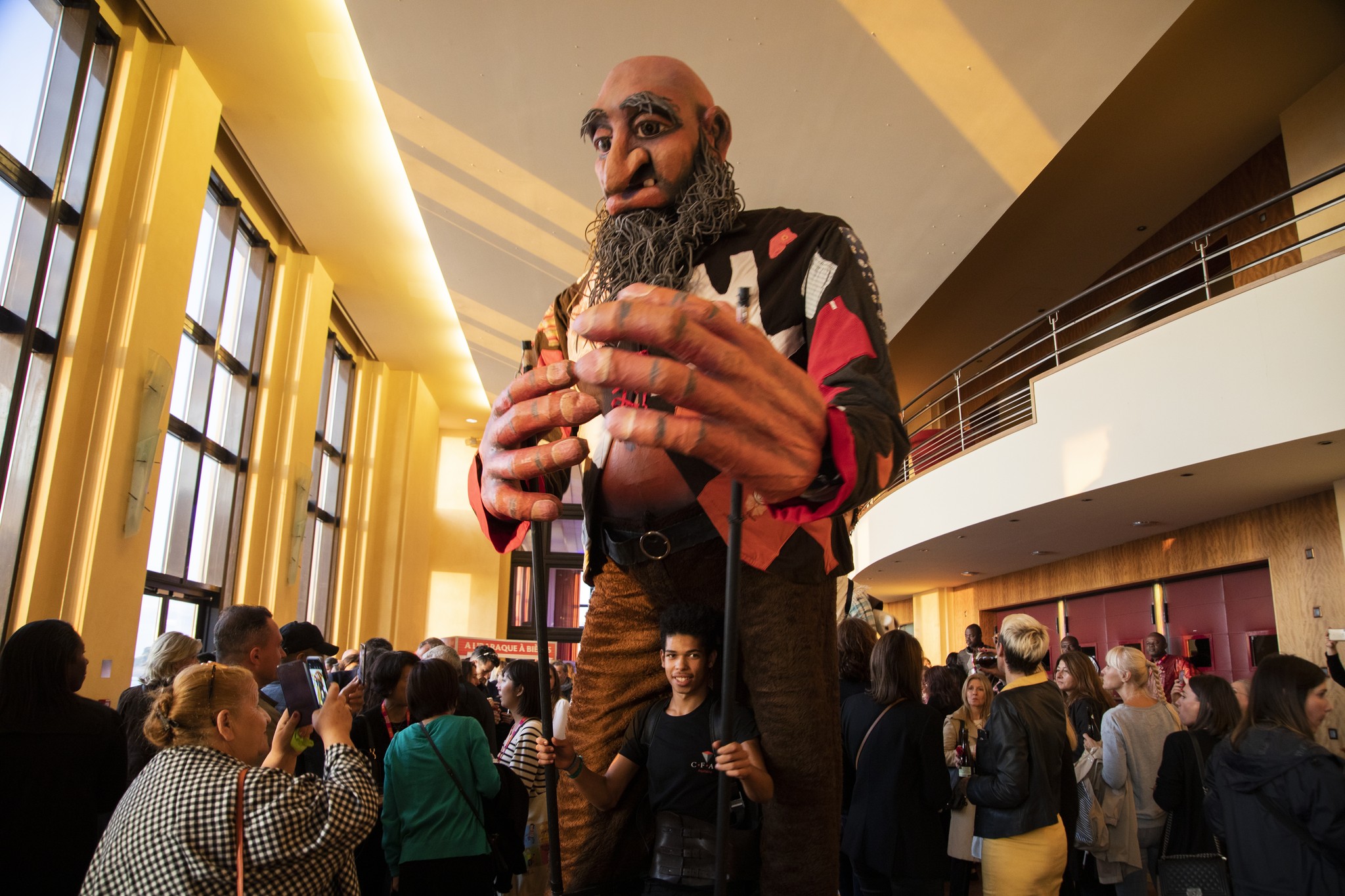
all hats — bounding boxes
[279,621,340,656]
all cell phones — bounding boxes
[358,643,367,686]
[977,728,987,741]
[1328,628,1345,641]
[306,656,330,708]
[1178,670,1184,689]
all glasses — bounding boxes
[475,651,498,660]
[992,633,999,644]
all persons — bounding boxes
[0,604,1345,895]
[466,56,910,893]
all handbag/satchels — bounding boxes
[947,720,967,810]
[488,834,508,872]
[1157,853,1229,896]
[357,714,382,789]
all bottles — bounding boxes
[973,651,998,669]
[1086,706,1101,742]
[959,728,975,780]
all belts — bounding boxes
[602,513,720,566]
